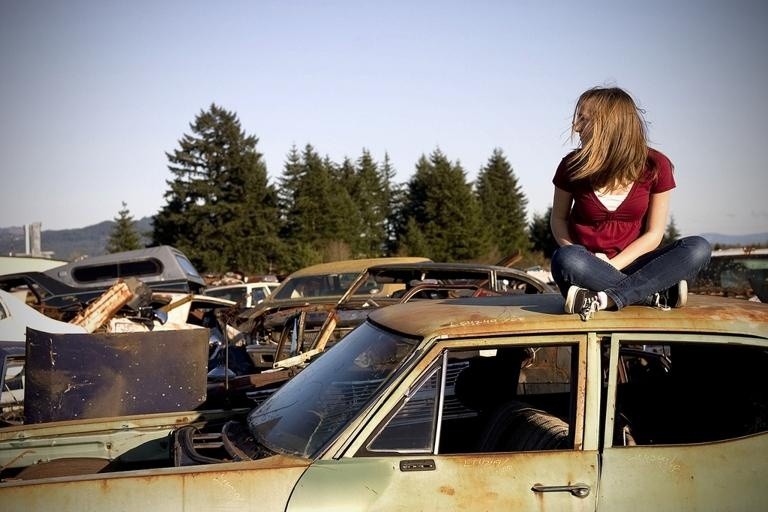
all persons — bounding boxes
[546,87,713,316]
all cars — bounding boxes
[0,292,768,512]
[0,250,568,427]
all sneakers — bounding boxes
[564,285,601,314]
[653,280,688,308]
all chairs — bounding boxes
[475,397,568,451]
